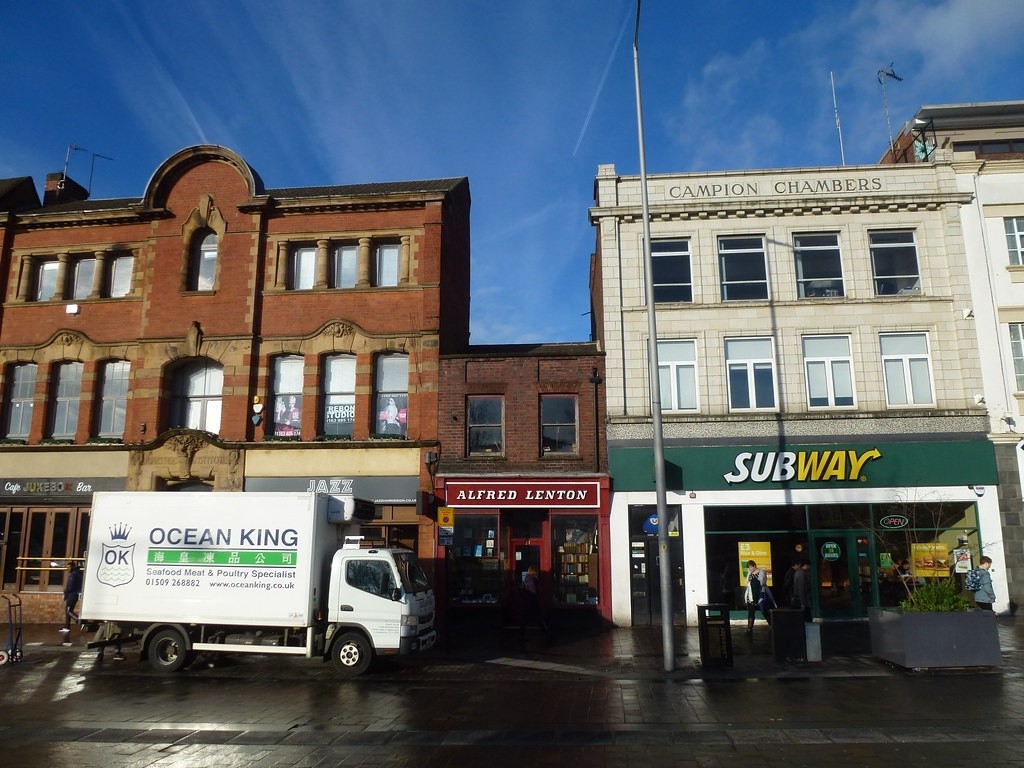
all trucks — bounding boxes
[77,491,438,678]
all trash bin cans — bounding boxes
[696,602,735,670]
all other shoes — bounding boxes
[113,653,126,660]
[59,628,70,633]
[80,623,84,631]
[95,654,104,662]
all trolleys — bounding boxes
[0,594,43,666]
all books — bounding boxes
[561,528,599,604]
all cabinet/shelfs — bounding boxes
[556,524,599,605]
[448,527,498,559]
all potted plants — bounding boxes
[867,577,1003,669]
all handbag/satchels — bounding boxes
[758,593,775,614]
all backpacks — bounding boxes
[964,569,987,590]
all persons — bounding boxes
[520,563,553,633]
[58,562,85,631]
[786,559,812,622]
[94,622,134,661]
[893,559,912,575]
[744,560,777,632]
[973,556,996,610]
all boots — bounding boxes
[745,618,756,633]
[765,615,771,629]
